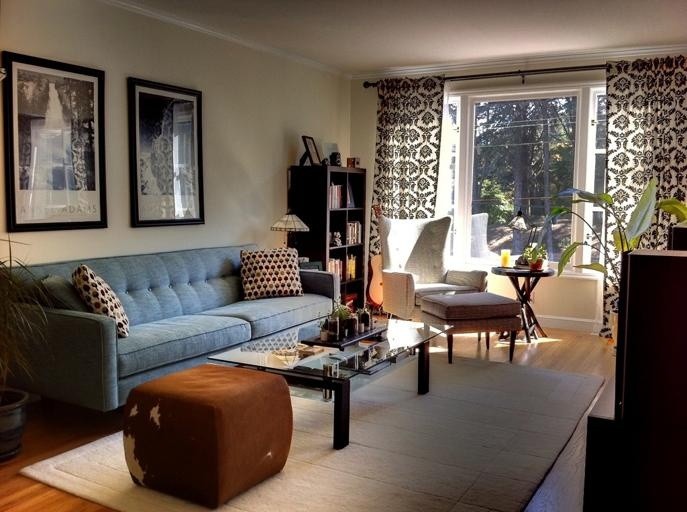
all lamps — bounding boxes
[271,207,310,249]
[507,209,537,269]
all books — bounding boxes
[336,355,391,375]
[329,183,361,283]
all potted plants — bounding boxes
[541,174,659,352]
[522,243,548,271]
[0,234,56,461]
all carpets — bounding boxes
[19,349,606,512]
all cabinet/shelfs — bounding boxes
[285,164,367,311]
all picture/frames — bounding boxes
[4,50,205,230]
[301,135,322,167]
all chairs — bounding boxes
[418,291,522,365]
[377,213,488,325]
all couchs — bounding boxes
[1,243,341,416]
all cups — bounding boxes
[500,248,511,268]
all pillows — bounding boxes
[41,263,130,340]
[239,247,305,300]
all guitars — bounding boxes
[367,204,383,308]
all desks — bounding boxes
[491,266,555,344]
